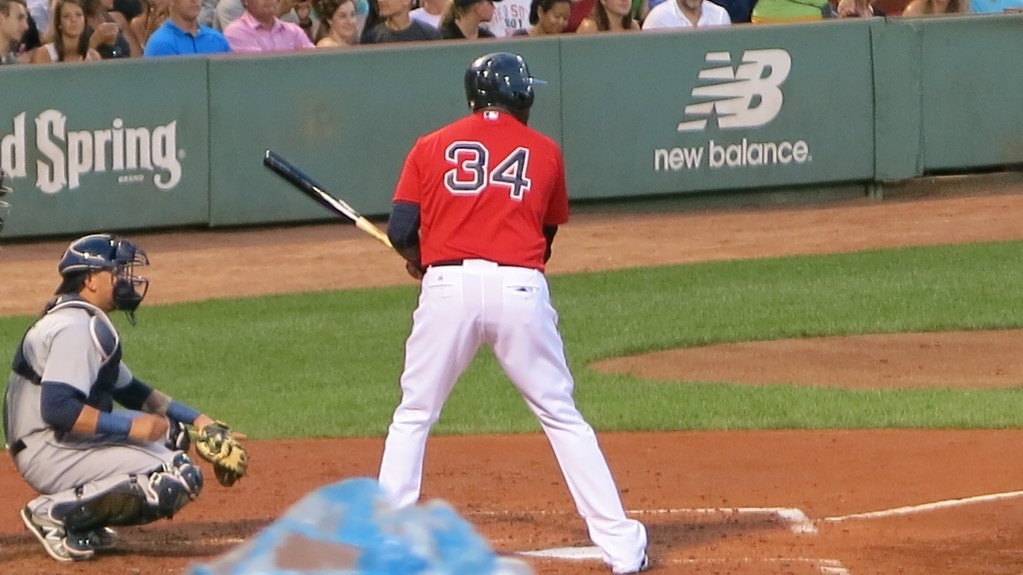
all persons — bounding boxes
[821,0,970,19]
[0,0,759,64]
[2,234,250,561]
[374,52,648,574]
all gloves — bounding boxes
[160,414,191,454]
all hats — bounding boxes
[52,234,119,296]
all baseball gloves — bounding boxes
[194,420,249,487]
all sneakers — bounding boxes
[83,526,118,550]
[20,495,95,561]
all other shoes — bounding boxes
[616,554,649,573]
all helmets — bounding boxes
[464,51,547,109]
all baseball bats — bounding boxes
[262,149,396,251]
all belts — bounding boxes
[426,259,516,266]
[8,439,25,456]
